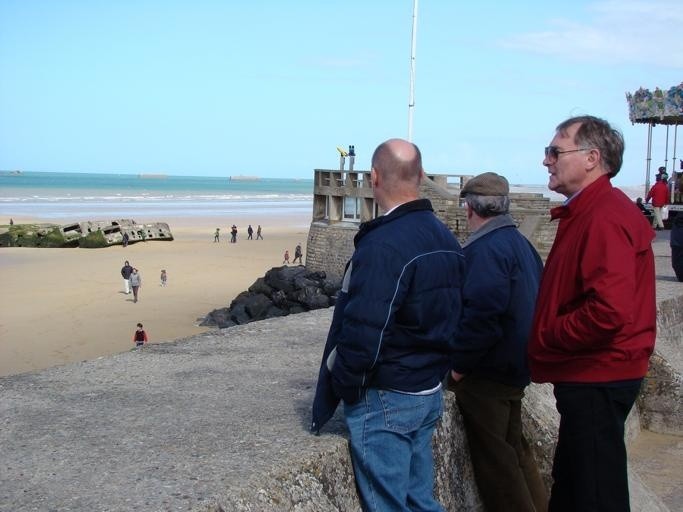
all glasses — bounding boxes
[544,146,589,164]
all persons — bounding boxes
[311,138,470,512]
[214,229,220,242]
[120,260,131,292]
[246,225,253,240]
[283,249,289,264]
[255,225,264,240]
[527,117,659,511]
[132,323,146,347]
[127,267,140,302]
[122,232,129,247]
[230,225,235,242]
[160,268,167,288]
[645,174,670,230]
[9,219,13,225]
[140,230,146,241]
[656,166,668,184]
[447,169,553,511]
[232,227,237,243]
[292,243,301,263]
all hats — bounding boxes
[459,172,508,198]
